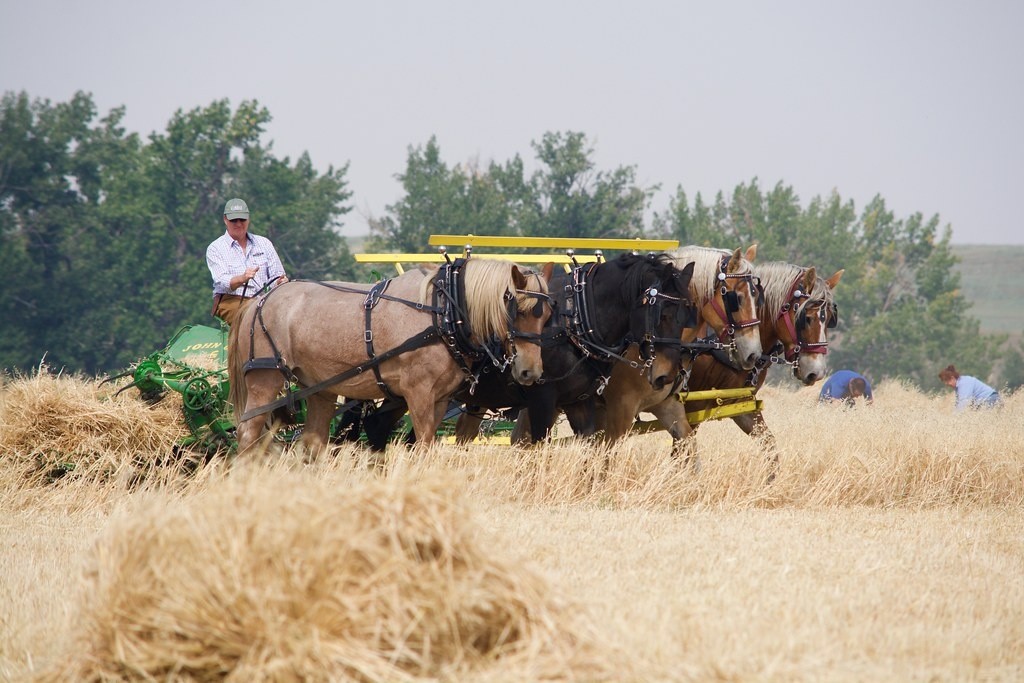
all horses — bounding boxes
[225,241,846,488]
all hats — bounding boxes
[223,198,249,220]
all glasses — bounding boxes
[229,218,246,223]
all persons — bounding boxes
[816,369,873,411]
[938,366,1004,418]
[205,198,289,327]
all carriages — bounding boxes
[97,232,846,489]
